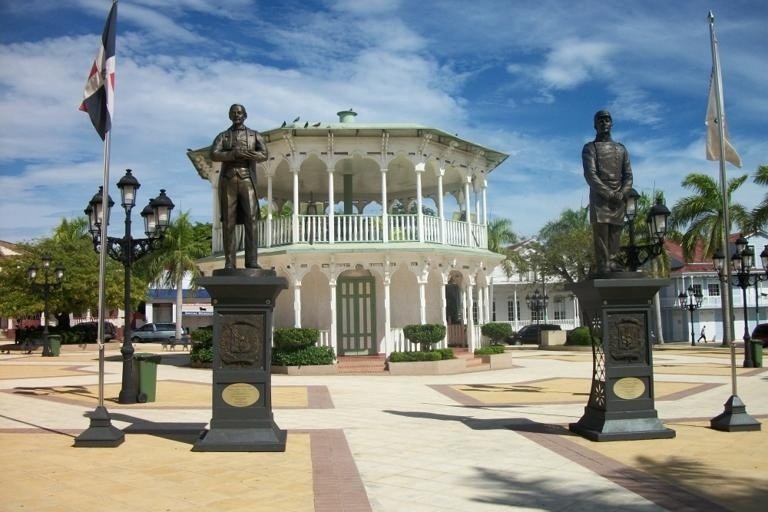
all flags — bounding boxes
[704,12,744,170]
[77,0,119,142]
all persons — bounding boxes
[697,325,709,343]
[208,103,269,269]
[581,110,634,273]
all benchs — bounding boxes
[159,336,192,353]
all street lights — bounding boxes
[712,233,768,368]
[525,288,549,344]
[83,167,176,406]
[28,252,67,357]
[585,186,672,273]
[678,284,704,346]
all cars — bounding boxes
[72,321,117,343]
[130,322,188,343]
[504,324,561,346]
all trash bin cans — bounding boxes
[750,340,763,368]
[47,335,61,357]
[133,353,161,403]
